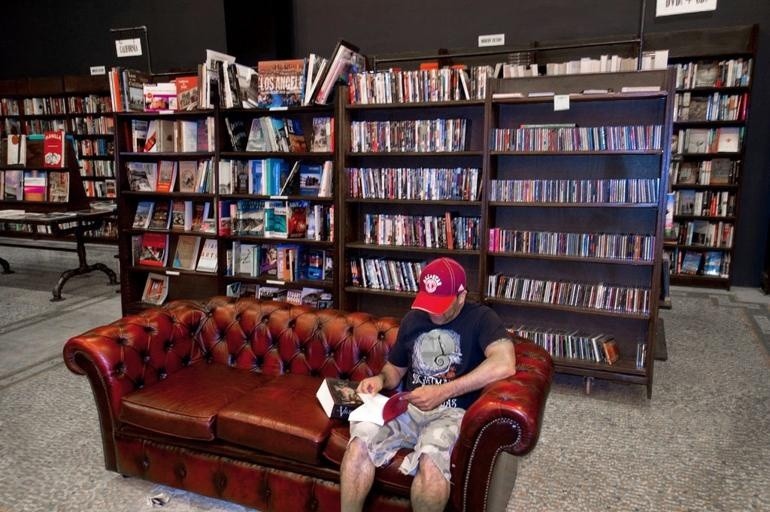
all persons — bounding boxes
[340,257,517,512]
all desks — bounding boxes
[0,202,121,302]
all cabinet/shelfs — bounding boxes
[23,70,91,246]
[481,67,682,403]
[338,58,486,323]
[1,74,34,245]
[62,67,127,251]
[108,55,225,320]
[654,49,758,293]
[209,57,344,316]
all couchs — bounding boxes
[60,292,557,512]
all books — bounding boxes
[218,116,335,311]
[487,271,652,315]
[347,64,495,105]
[112,41,368,113]
[506,322,619,366]
[656,260,672,309]
[344,166,483,202]
[125,116,218,304]
[348,392,413,425]
[668,59,751,88]
[491,123,662,152]
[2,130,117,240]
[349,257,428,293]
[2,98,115,198]
[350,120,467,152]
[487,179,660,204]
[489,228,656,262]
[636,341,648,366]
[675,93,749,124]
[493,49,671,78]
[315,377,391,421]
[364,213,480,250]
[664,128,744,277]
[491,87,661,112]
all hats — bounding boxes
[411,257,467,315]
